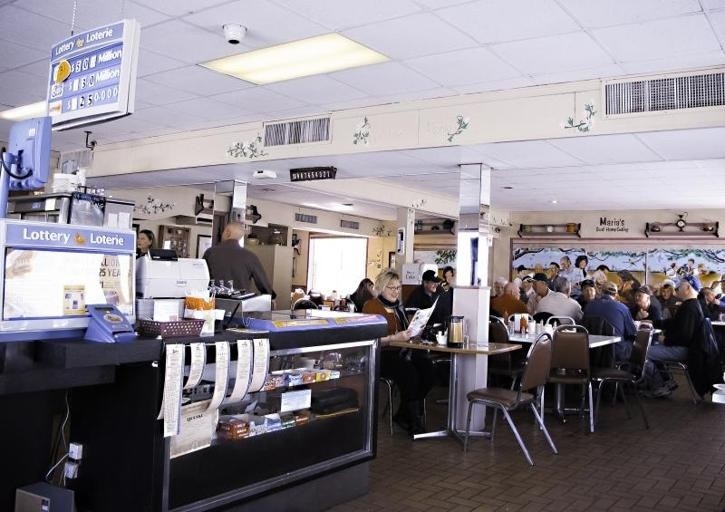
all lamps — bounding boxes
[592,323,653,430]
[463,332,559,466]
[292,298,319,310]
[657,360,704,405]
[539,324,594,433]
[378,374,426,438]
[489,314,530,420]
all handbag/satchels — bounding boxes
[389,336,393,342]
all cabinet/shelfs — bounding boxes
[520,316,527,334]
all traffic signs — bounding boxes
[63,463,78,478]
[69,442,83,460]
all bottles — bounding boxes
[581,279,594,288]
[528,273,548,283]
[684,275,702,292]
[601,280,618,293]
[634,285,651,296]
[423,270,443,283]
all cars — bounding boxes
[347,303,355,313]
[506,322,514,337]
[528,320,535,334]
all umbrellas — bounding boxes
[392,415,410,432]
[411,417,425,437]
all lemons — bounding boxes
[447,315,464,349]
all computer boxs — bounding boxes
[224,24,247,45]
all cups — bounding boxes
[386,286,401,291]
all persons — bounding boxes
[528,263,544,278]
[346,278,374,313]
[698,287,725,321]
[654,279,681,321]
[663,258,706,291]
[202,221,280,313]
[526,274,554,314]
[576,280,598,310]
[547,262,557,290]
[627,287,659,324]
[136,228,155,257]
[579,282,636,400]
[362,268,438,442]
[569,256,588,298]
[402,270,440,339]
[534,278,585,328]
[592,264,608,299]
[512,266,523,292]
[617,270,640,310]
[638,274,705,399]
[496,269,533,323]
[437,266,453,329]
[559,254,570,292]
[291,288,319,309]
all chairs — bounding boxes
[643,384,672,399]
[666,378,678,387]
